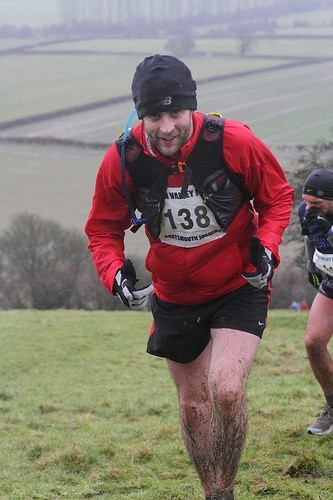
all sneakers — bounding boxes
[306,403,333,436]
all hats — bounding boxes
[130,53,198,120]
[302,168,333,201]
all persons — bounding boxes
[290,299,307,311]
[86,54,294,500]
[298,169,333,436]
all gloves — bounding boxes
[301,206,330,236]
[112,259,155,311]
[240,237,274,291]
